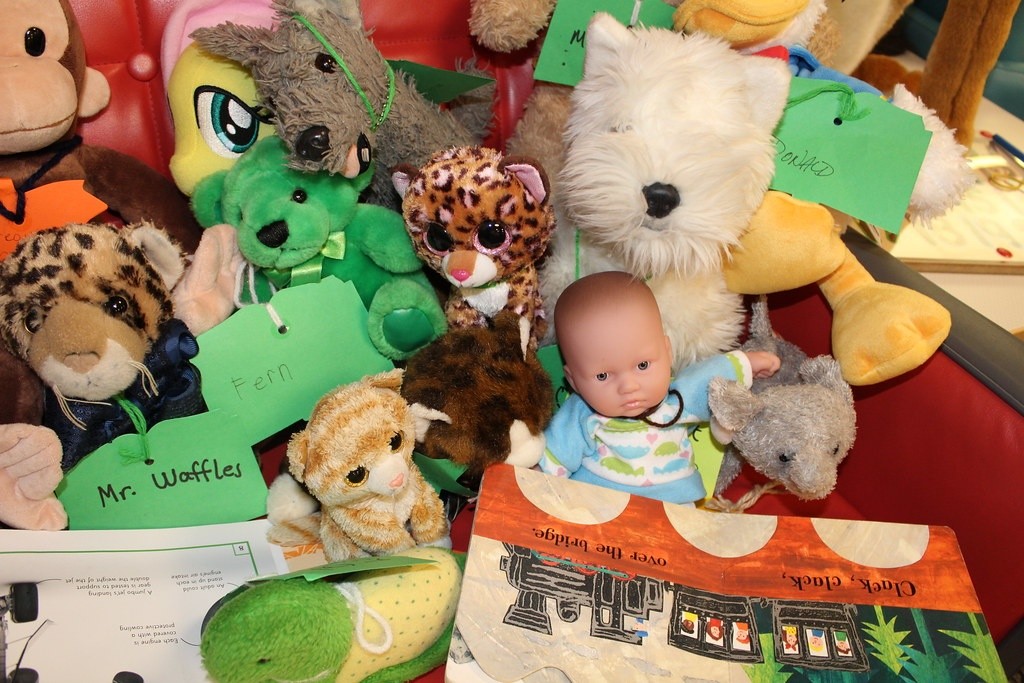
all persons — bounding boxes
[536,271,781,510]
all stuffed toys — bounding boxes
[0,0,973,683]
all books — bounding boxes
[443,465,1010,683]
[888,153,1023,267]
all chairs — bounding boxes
[69,0,1024,683]
